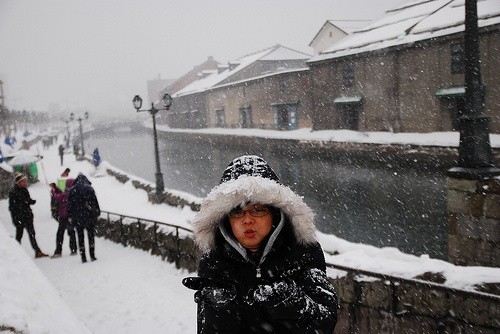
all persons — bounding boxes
[68,172,101,263]
[8,174,49,258]
[92,148,100,166]
[59,146,65,160]
[182,155,339,334]
[49,183,62,195]
[52,193,77,259]
[62,168,70,177]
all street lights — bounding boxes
[132,92,173,190]
[69,111,90,157]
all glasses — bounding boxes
[228,207,274,219]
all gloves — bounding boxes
[242,277,294,311]
[182,277,237,308]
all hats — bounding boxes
[60,167,70,175]
[14,172,27,184]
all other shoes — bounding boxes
[50,254,62,259]
[36,252,49,258]
[69,252,77,256]
